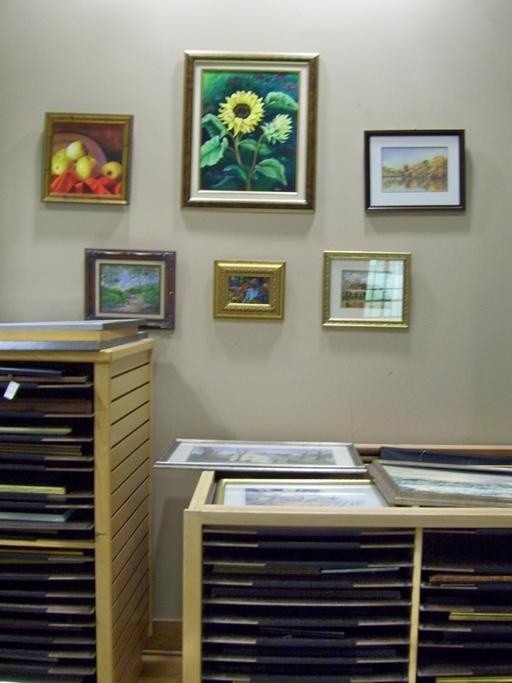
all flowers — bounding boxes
[198,82,298,193]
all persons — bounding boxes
[234,277,269,304]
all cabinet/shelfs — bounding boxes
[1,335,159,681]
[178,441,509,679]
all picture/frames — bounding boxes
[41,110,132,206]
[212,259,285,325]
[179,48,318,215]
[362,128,466,217]
[320,251,411,331]
[82,246,176,332]
[153,432,511,512]
